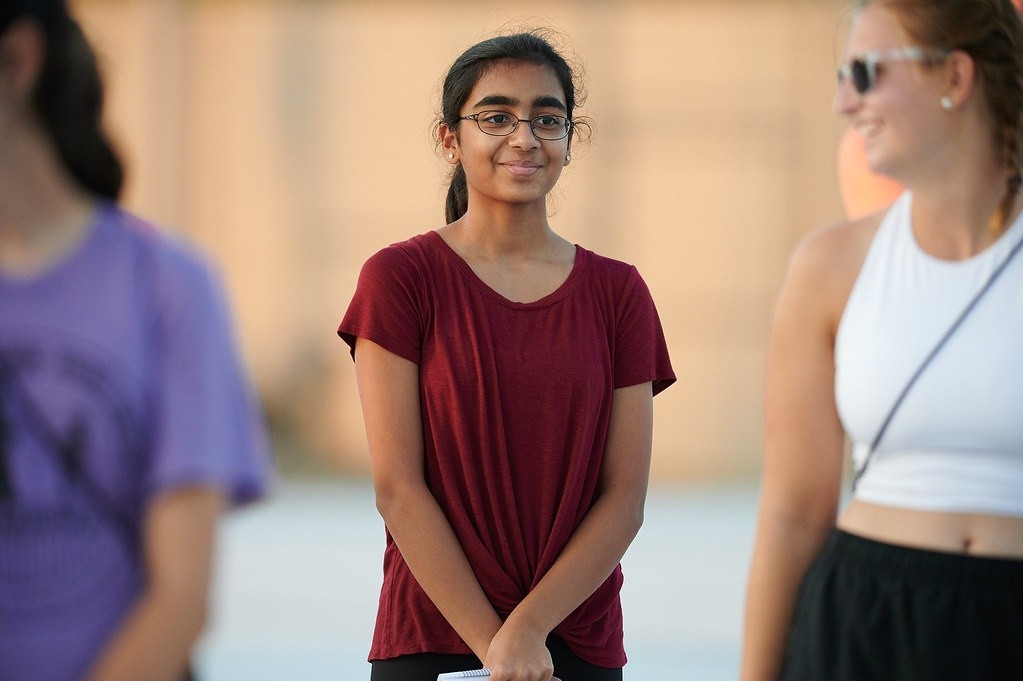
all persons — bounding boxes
[740,0,1023,680]
[337,33,675,680]
[0,0,271,681]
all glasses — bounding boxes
[459,110,575,140]
[837,47,950,93]
